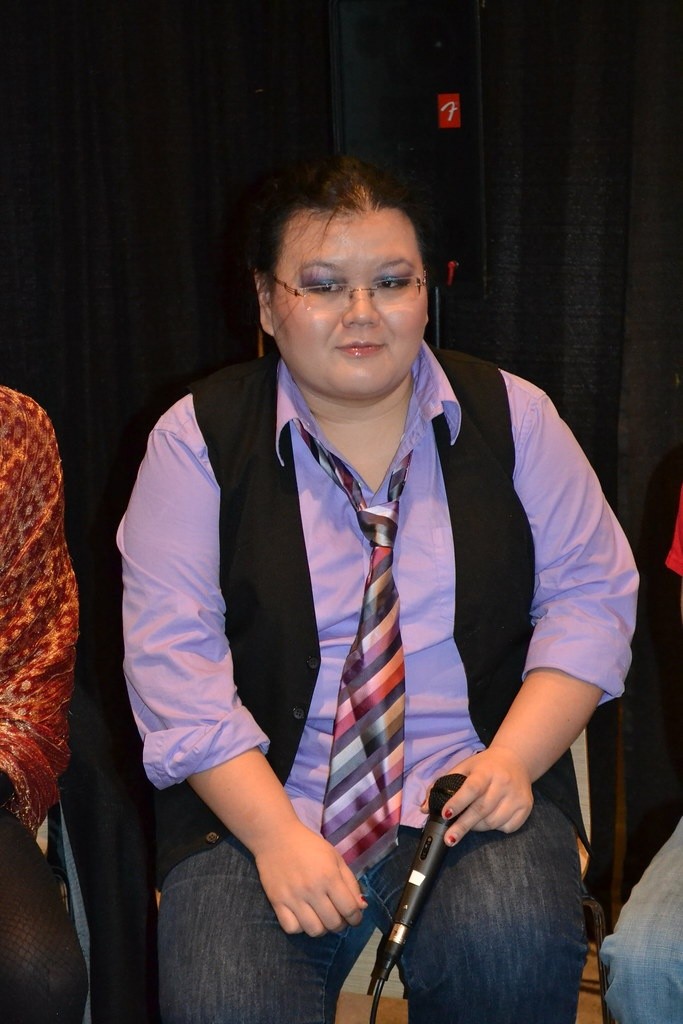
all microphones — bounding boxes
[378,773,467,981]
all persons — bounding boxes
[115,156,641,1024]
[597,483,682,1024]
[0,386,87,1024]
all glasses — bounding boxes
[271,271,426,313]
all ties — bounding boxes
[291,415,415,886]
